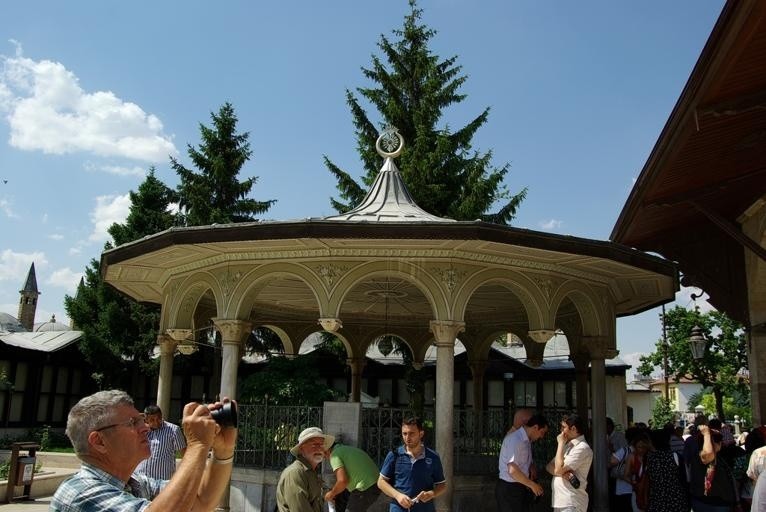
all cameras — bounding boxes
[210,401,238,428]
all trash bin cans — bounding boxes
[4,441,41,504]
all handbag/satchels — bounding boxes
[635,472,648,511]
[610,462,627,479]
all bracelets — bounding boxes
[210,453,234,465]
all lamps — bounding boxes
[686,291,709,361]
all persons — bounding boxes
[496,415,548,511]
[134,406,187,481]
[271,427,334,512]
[323,443,382,512]
[546,412,594,512]
[376,418,446,512]
[604,416,766,511]
[505,410,533,438]
[46,389,238,512]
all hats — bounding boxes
[290,427,335,458]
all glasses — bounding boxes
[299,442,327,450]
[94,413,144,431]
